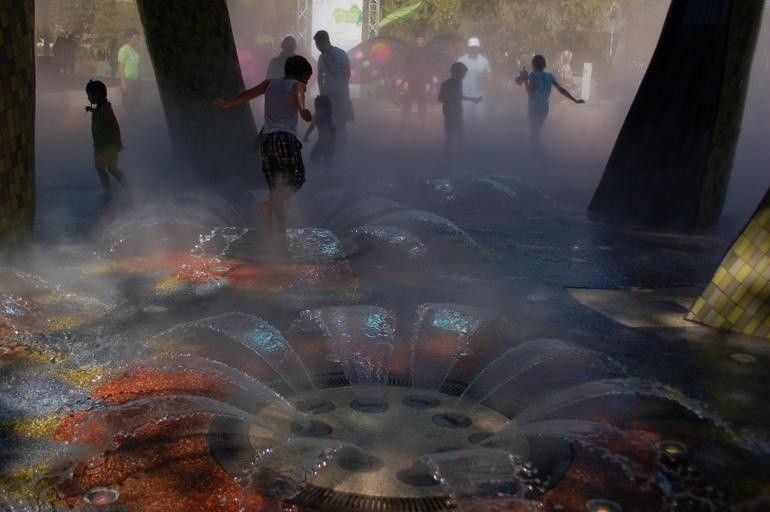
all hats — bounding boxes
[467,36,479,48]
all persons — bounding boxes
[561,47,579,97]
[522,53,585,159]
[393,35,438,128]
[83,79,134,200]
[221,56,313,235]
[458,36,491,128]
[117,28,141,116]
[266,28,354,168]
[49,33,77,79]
[440,63,483,151]
